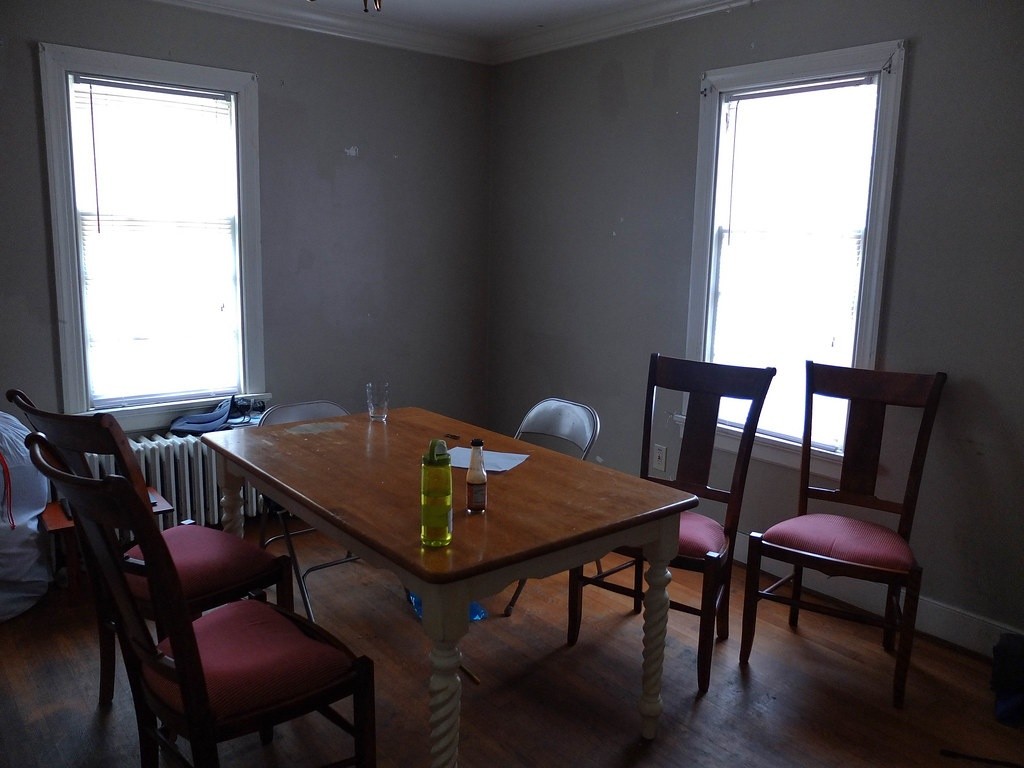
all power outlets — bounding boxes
[654,444,666,472]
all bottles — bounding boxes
[466,439,487,513]
[421,439,453,548]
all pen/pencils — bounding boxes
[461,665,481,683]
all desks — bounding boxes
[199,407,703,768]
[40,486,174,593]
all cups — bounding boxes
[366,382,390,422]
[367,421,387,460]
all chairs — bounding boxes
[504,397,601,619]
[23,433,378,768]
[739,360,947,708]
[5,386,294,743]
[567,353,775,692]
[260,400,366,625]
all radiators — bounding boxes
[81,429,265,531]
